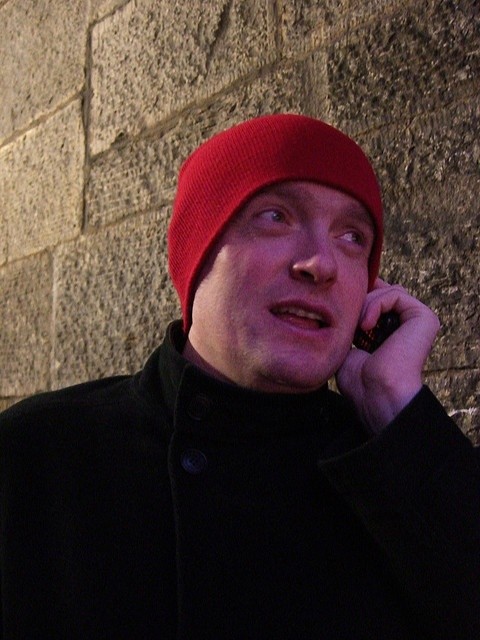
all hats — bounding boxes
[167,113,384,332]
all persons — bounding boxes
[1,110,480,640]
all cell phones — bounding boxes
[351,304,402,354]
